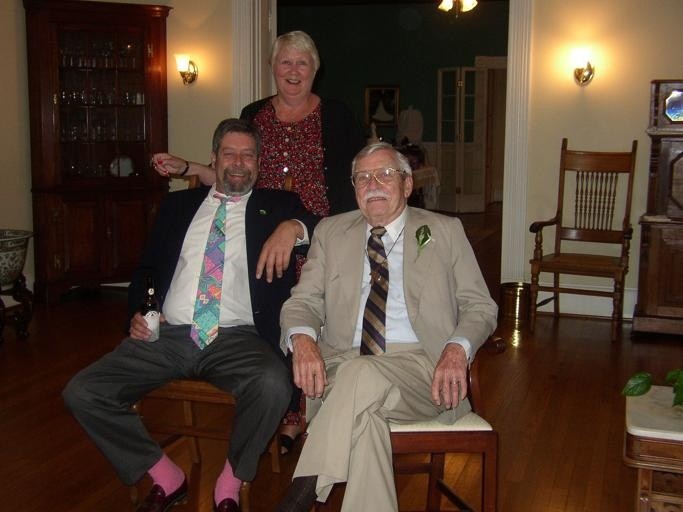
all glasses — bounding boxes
[350,167,406,190]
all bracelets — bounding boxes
[179,160,190,177]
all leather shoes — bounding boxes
[278,483,317,512]
[135,471,188,512]
[212,487,242,512]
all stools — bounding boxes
[0,276,34,340]
[386,333,507,511]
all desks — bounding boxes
[620,383,682,511]
[410,164,438,208]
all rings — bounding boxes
[153,165,156,170]
[449,380,459,384]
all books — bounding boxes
[641,213,672,223]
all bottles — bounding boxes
[140,275,161,344]
[60,41,137,68]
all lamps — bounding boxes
[173,54,197,88]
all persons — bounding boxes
[152,29,356,458]
[281,142,499,512]
[60,117,327,512]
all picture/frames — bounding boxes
[364,86,400,129]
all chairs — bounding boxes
[526,135,638,342]
[127,175,292,512]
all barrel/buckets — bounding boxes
[502,282,531,322]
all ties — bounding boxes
[360,226,389,356]
[189,193,241,352]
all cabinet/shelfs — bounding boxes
[631,78,682,339]
[22,0,171,307]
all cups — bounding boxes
[57,86,134,105]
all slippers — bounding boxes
[267,430,304,457]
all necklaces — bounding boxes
[365,226,407,284]
